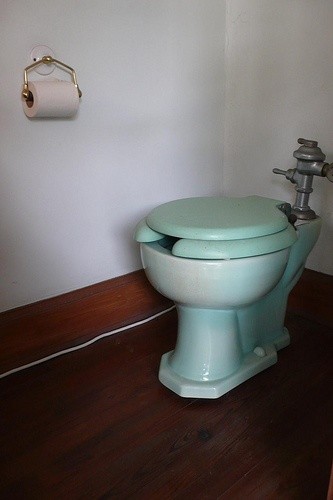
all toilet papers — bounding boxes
[23,80,80,119]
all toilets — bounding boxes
[134,194,323,400]
